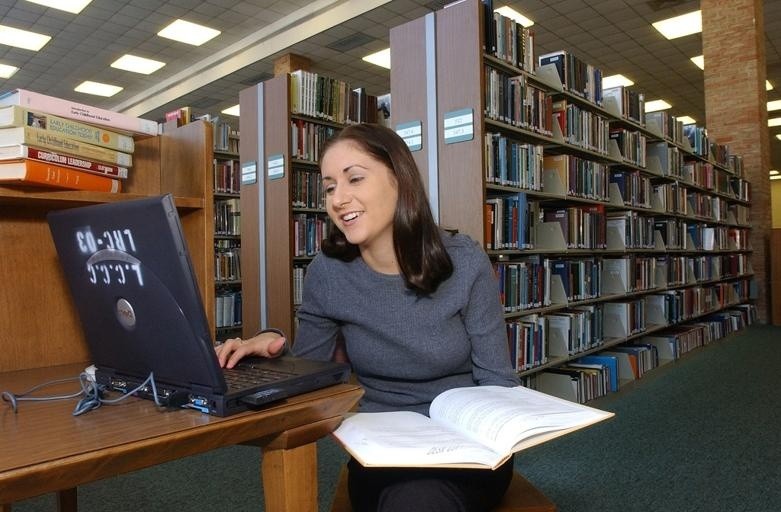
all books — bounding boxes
[289,71,393,353]
[0,85,162,191]
[483,0,756,402]
[333,387,614,474]
[168,106,240,335]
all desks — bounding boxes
[0,119,366,512]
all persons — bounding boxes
[216,123,516,509]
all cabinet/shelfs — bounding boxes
[387,1,758,405]
[159,120,241,342]
[237,74,391,354]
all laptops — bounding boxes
[47,193,350,416]
